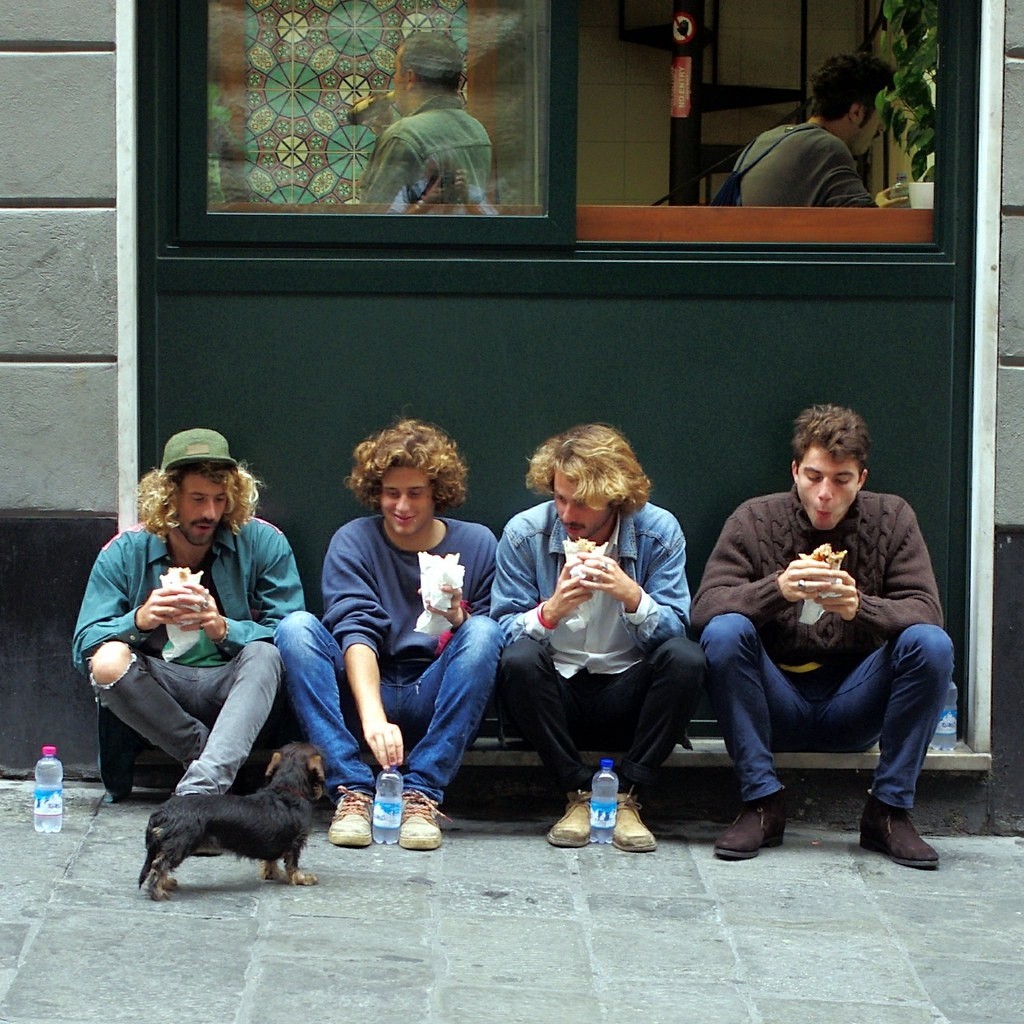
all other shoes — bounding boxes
[194,835,223,854]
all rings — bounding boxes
[798,579,804,591]
[601,561,607,572]
[201,588,209,598]
[201,598,208,609]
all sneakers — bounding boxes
[328,785,372,845]
[713,786,786,858]
[547,789,592,847]
[612,793,657,851]
[399,788,442,849]
[860,788,939,866]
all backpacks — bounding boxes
[709,126,818,207]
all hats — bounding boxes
[160,428,236,472]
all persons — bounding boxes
[273,419,506,851]
[690,405,955,868]
[383,147,499,215]
[73,429,306,797]
[732,51,909,209]
[490,424,709,853]
[353,31,492,205]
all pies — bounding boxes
[562,536,598,559]
[808,544,848,571]
[159,566,203,583]
[418,551,460,566]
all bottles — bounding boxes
[889,171,912,208]
[371,765,405,845]
[589,759,619,843]
[34,745,66,834]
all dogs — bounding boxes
[138,742,326,902]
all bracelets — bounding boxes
[200,622,203,629]
[210,615,228,645]
[451,608,468,633]
[537,601,560,629]
